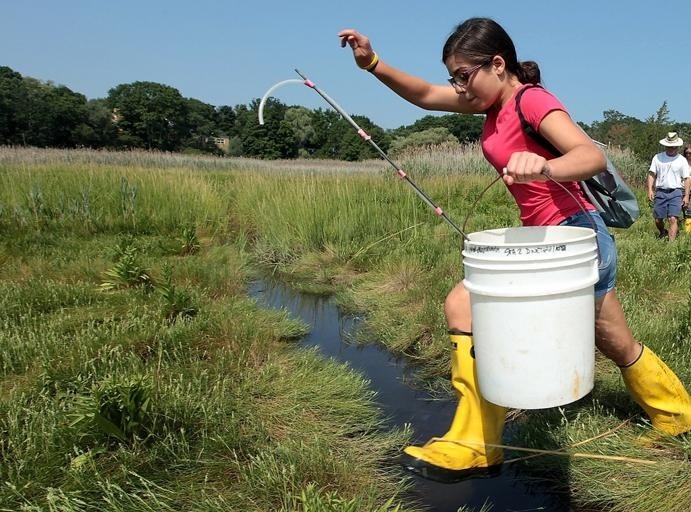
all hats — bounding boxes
[659,132,683,147]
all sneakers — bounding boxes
[659,229,668,239]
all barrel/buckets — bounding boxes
[464,173,601,411]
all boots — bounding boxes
[400,331,506,484]
[615,342,691,449]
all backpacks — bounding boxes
[515,85,640,229]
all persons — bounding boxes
[684,143,691,164]
[646,131,691,245]
[336,15,691,485]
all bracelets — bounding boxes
[357,51,380,71]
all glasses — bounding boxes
[447,63,486,88]
